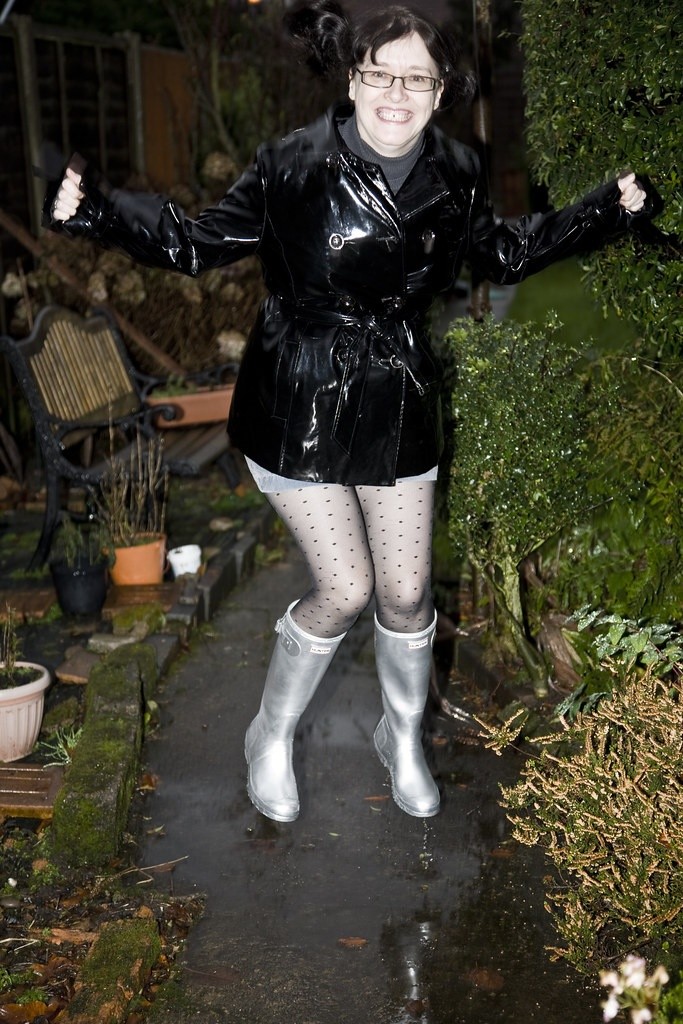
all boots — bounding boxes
[245,599,363,823]
[373,600,440,816]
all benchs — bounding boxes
[3,306,245,576]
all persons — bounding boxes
[41,0,662,823]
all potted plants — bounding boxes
[147,373,237,427]
[98,429,169,585]
[0,601,51,764]
[48,510,117,618]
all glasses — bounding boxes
[352,65,442,92]
